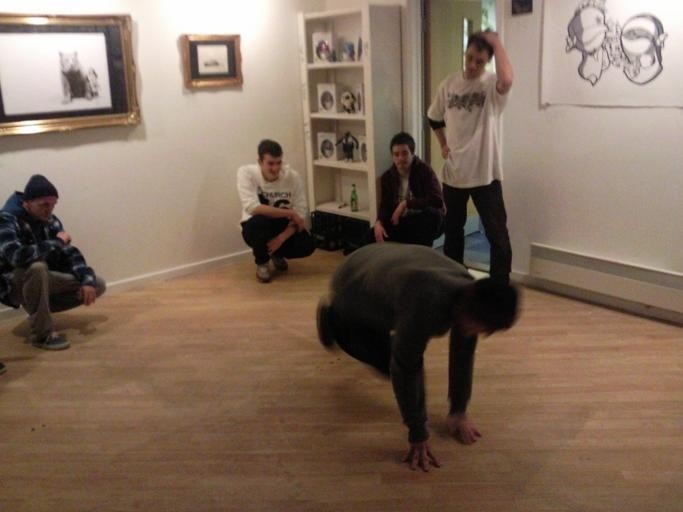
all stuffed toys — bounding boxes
[340,89,356,114]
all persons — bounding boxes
[428,27,511,285]
[315,241,522,475]
[236,139,317,282]
[319,41,328,60]
[369,133,448,247]
[1,175,106,351]
[335,129,359,165]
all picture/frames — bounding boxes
[179,33,246,91]
[0,13,145,138]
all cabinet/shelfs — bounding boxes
[297,5,405,231]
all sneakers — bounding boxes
[33,336,69,350]
[270,254,287,270]
[255,263,269,281]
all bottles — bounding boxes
[349,183,358,213]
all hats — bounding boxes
[24,175,57,198]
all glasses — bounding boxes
[27,201,58,211]
